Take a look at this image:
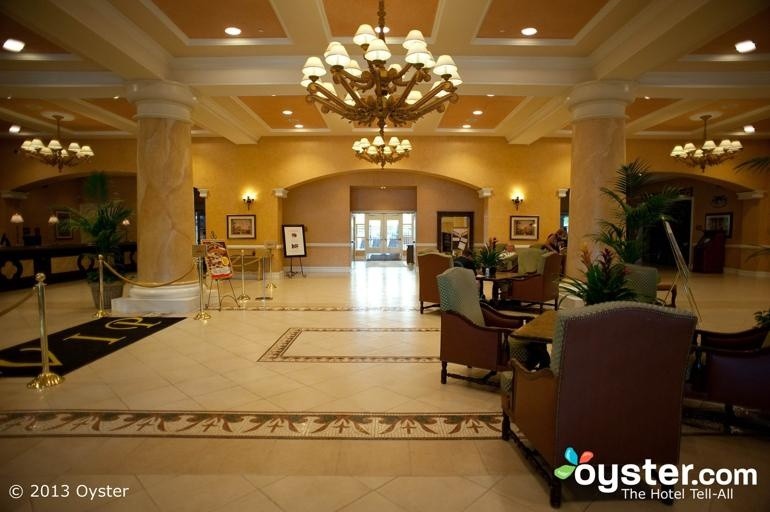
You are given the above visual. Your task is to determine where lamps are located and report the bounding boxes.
[243,192,255,211]
[122,219,130,243]
[511,192,524,211]
[298,0,462,132]
[21,112,94,173]
[670,115,743,173]
[351,120,412,169]
[47,215,59,224]
[9,213,24,247]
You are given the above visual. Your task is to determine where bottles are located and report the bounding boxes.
[486,268,489,276]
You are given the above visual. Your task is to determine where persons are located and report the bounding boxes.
[454,249,477,276]
[497,243,518,265]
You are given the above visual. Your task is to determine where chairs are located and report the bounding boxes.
[417,242,770,508]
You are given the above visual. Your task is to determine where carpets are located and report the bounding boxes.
[0,317,187,378]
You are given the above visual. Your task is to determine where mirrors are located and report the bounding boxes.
[437,211,474,253]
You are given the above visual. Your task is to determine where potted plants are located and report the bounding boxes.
[49,169,134,310]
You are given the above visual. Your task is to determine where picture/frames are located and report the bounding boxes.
[510,215,539,240]
[201,239,233,279]
[227,214,255,239]
[705,211,733,239]
[55,210,73,240]
[282,225,306,257]
